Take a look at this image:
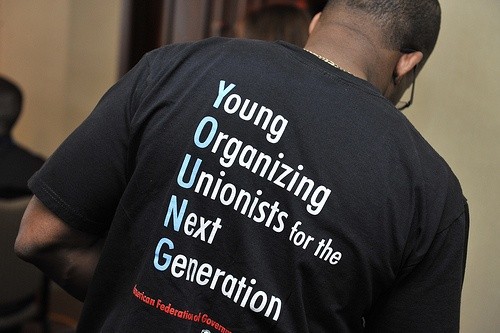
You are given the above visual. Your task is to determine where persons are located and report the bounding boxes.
[13,0,471,333]
[0,73,45,200]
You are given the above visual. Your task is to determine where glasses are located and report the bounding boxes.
[395,65,416,110]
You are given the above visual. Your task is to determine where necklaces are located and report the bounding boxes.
[301,47,355,76]
